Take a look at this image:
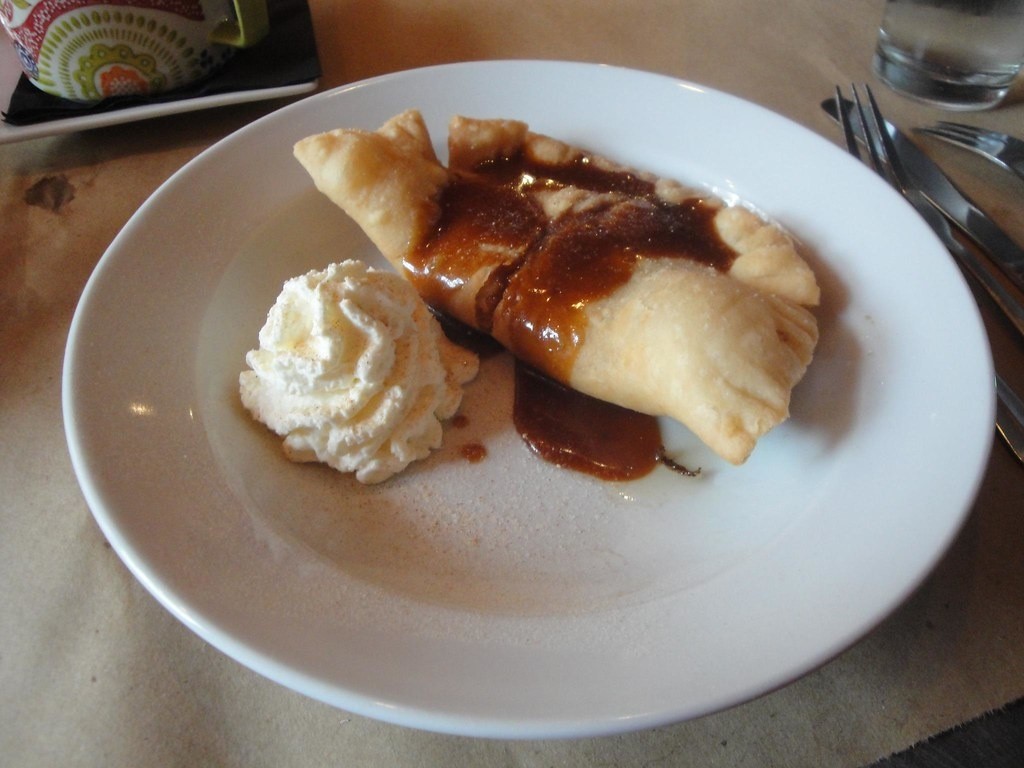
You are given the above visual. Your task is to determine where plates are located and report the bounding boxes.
[63,58,997,740]
[0,0,320,144]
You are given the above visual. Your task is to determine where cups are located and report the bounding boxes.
[871,0,1024,112]
[0,0,268,104]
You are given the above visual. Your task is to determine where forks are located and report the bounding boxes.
[909,121,1024,183]
[835,84,1024,464]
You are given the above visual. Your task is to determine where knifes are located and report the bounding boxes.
[817,99,1023,295]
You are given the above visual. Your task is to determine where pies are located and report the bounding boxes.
[293,109,822,464]
